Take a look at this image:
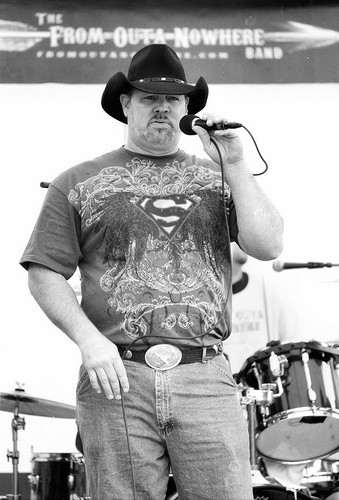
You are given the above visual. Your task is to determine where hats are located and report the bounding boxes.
[101,44,208,125]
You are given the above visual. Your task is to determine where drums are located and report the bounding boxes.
[29,450,86,500]
[238,341,339,465]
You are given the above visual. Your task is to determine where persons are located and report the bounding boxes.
[19,43,284,500]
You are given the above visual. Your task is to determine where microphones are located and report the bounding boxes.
[273,259,332,272]
[180,114,242,135]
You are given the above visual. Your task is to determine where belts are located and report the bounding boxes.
[118,345,219,371]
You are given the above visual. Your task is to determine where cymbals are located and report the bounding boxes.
[0,392,77,418]
